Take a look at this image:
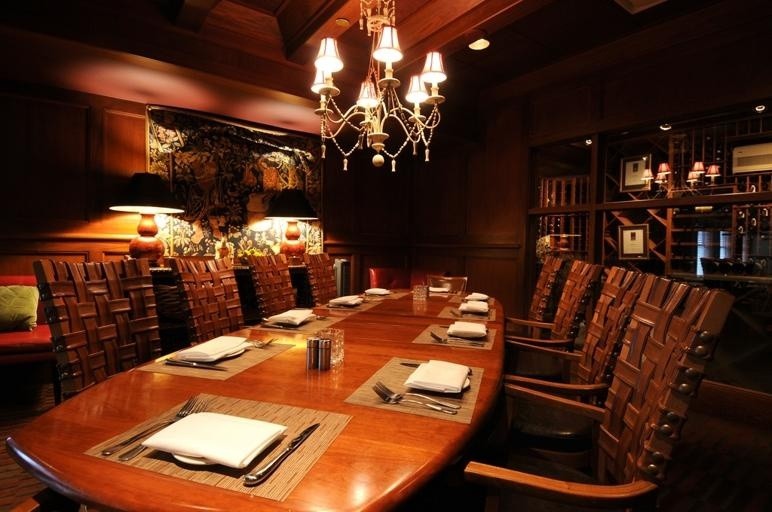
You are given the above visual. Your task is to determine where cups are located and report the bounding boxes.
[316,328,346,365]
[413,284,429,301]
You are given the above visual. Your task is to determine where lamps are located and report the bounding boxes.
[308,1,450,174]
[643,133,725,201]
[108,174,185,264]
[261,189,323,266]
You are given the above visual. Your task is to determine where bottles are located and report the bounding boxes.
[305,335,332,369]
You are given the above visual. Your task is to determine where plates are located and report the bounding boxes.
[462,376,470,388]
[170,451,215,467]
[219,347,245,359]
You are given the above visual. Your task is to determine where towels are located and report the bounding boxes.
[175,335,254,366]
[445,320,488,338]
[322,287,489,315]
[259,302,318,330]
[139,413,289,470]
[402,357,472,398]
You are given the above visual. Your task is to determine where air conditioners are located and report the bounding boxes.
[729,143,771,175]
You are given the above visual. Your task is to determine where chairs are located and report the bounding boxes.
[165,253,248,338]
[1,272,66,421]
[37,255,163,401]
[242,253,298,330]
[425,273,468,293]
[299,255,343,307]
[462,253,736,512]
[368,265,451,294]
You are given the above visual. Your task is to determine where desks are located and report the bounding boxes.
[119,258,315,356]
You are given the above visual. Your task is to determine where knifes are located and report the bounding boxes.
[260,322,305,331]
[439,325,449,328]
[165,355,230,372]
[325,304,363,310]
[400,362,472,375]
[243,422,319,486]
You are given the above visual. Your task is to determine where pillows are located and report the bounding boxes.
[2,284,41,330]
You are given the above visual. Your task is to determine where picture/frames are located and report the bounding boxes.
[618,156,651,192]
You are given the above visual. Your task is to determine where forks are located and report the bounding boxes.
[449,309,490,320]
[372,380,461,415]
[430,330,484,345]
[101,392,200,455]
[119,396,208,462]
[247,336,279,349]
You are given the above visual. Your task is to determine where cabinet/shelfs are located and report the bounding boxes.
[602,172,772,280]
